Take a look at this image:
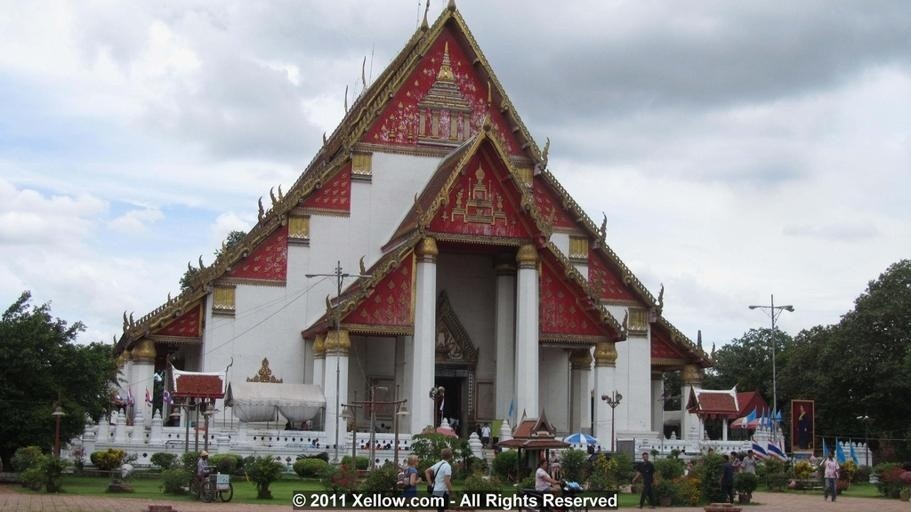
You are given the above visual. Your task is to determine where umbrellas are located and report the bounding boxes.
[563,431,598,451]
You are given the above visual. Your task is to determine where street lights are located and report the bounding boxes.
[601,390,622,452]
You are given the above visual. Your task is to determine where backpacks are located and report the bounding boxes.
[396,470,410,492]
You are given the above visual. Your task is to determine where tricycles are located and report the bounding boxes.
[188,466,233,503]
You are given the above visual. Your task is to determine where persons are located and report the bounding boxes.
[424,448,453,512]
[477,423,483,440]
[632,452,656,508]
[535,458,565,495]
[742,449,757,498]
[195,450,212,500]
[719,454,735,504]
[821,449,840,502]
[403,455,423,511]
[553,458,563,480]
[731,451,741,494]
[796,404,811,448]
[482,422,493,448]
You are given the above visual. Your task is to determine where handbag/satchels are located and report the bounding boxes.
[426,484,434,493]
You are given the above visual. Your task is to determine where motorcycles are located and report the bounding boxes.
[520,477,588,512]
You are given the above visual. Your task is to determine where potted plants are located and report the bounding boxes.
[38,454,67,493]
[656,478,673,506]
[72,446,86,470]
[732,472,757,505]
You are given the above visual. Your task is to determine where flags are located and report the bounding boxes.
[836,436,847,464]
[747,406,782,432]
[440,393,445,418]
[767,438,789,463]
[850,439,859,465]
[128,388,135,407]
[751,438,768,462]
[164,389,176,404]
[823,437,831,458]
[145,386,154,404]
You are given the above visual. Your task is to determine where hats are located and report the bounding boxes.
[200,450,208,457]
